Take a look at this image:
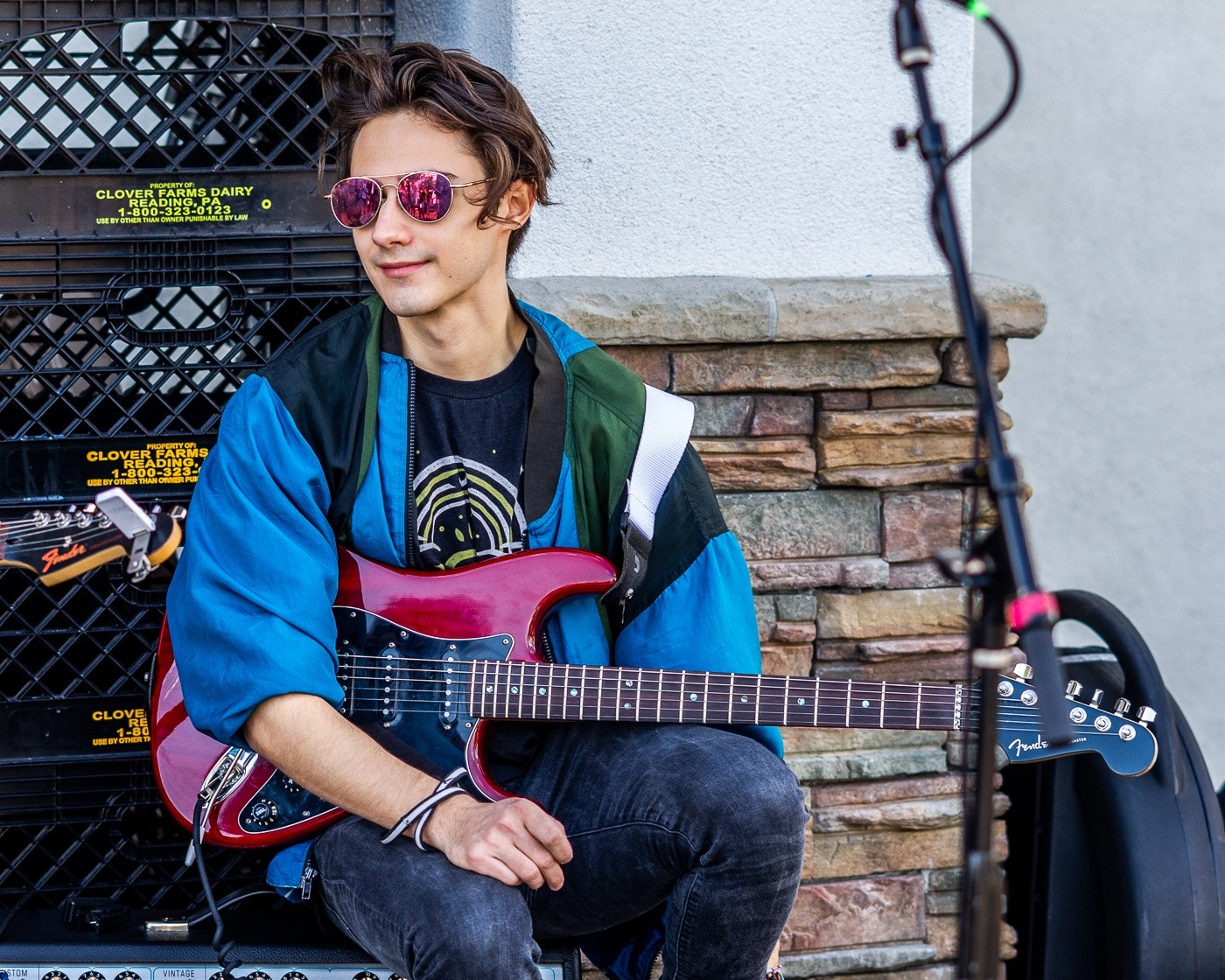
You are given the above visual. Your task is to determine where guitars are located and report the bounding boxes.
[149,544,1158,852]
[0,484,183,590]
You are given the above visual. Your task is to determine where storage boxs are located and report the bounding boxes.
[0,1,398,946]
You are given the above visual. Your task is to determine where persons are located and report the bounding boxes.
[165,41,810,980]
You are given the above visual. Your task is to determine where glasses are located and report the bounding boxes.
[324,171,498,229]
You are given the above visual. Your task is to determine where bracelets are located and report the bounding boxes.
[765,965,784,980]
[379,765,469,853]
